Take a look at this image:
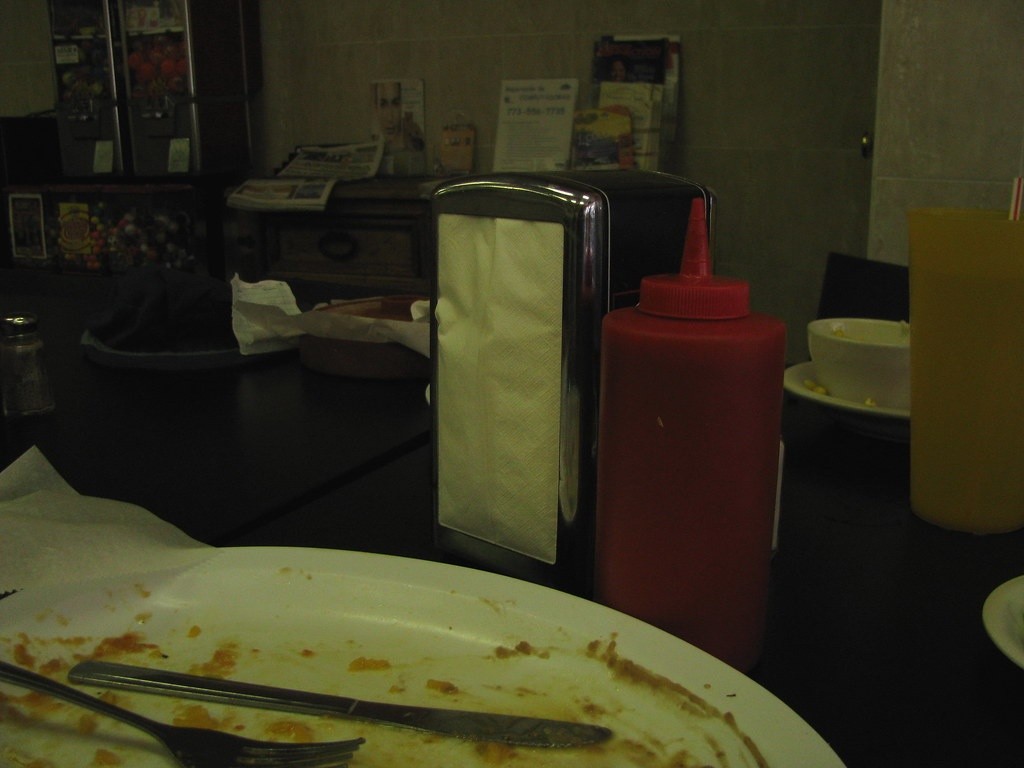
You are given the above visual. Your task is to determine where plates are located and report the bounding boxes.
[81,327,272,368]
[1,545,847,768]
[782,359,912,418]
[982,574,1024,672]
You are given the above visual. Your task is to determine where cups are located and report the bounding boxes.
[807,317,911,415]
[901,203,1024,538]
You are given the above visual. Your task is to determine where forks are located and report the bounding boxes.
[0,658,367,767]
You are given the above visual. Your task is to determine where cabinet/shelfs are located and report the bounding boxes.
[225,169,709,343]
[119,1,248,176]
[46,1,115,181]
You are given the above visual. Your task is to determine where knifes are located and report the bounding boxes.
[68,656,616,750]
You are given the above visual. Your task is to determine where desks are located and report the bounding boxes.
[1,261,1023,768]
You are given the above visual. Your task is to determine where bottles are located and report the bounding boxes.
[592,195,788,674]
[0,310,56,421]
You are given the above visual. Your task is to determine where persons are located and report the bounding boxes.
[371,82,406,155]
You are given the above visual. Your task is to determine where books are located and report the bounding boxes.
[492,35,683,177]
[437,122,476,177]
[371,77,427,177]
[227,140,383,210]
